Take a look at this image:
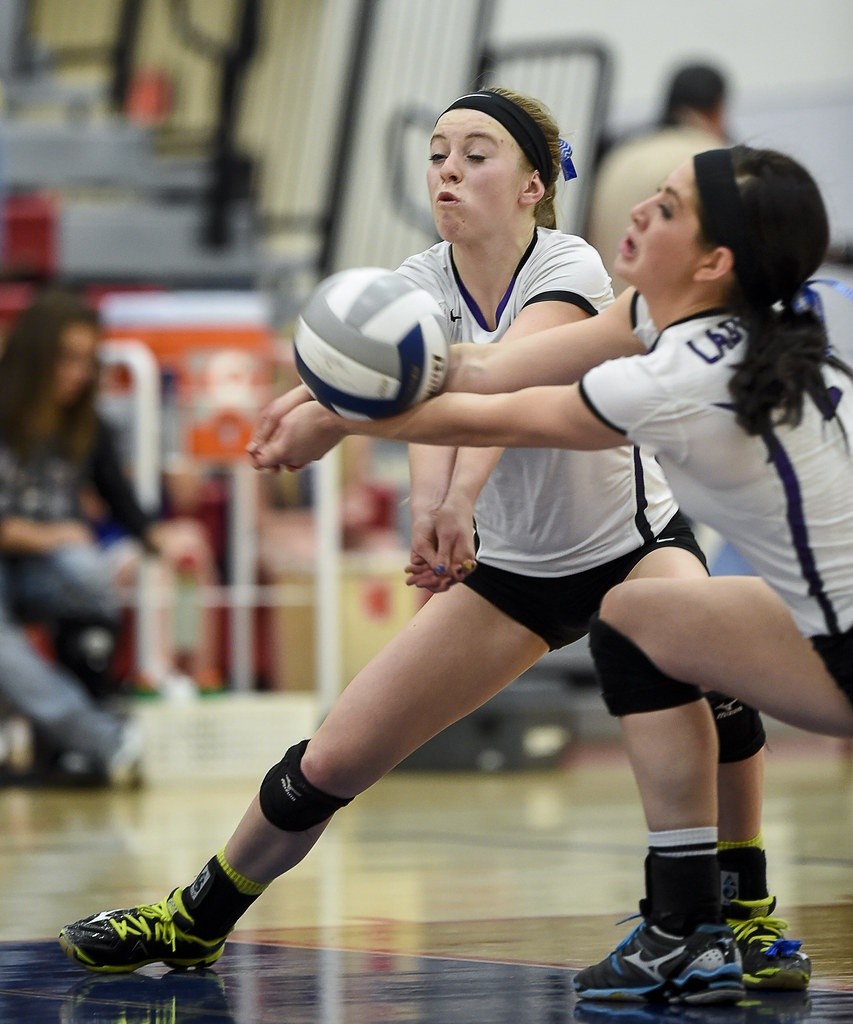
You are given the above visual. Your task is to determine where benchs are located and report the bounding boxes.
[0,80,410,559]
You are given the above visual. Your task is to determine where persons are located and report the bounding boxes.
[1,293,196,790]
[591,63,745,305]
[54,85,814,998]
[244,145,853,1012]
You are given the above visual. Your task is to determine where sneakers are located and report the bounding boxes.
[570,898,746,1006]
[720,896,812,994]
[60,970,227,1024]
[56,886,238,972]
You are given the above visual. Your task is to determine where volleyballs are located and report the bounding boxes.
[290,265,451,424]
